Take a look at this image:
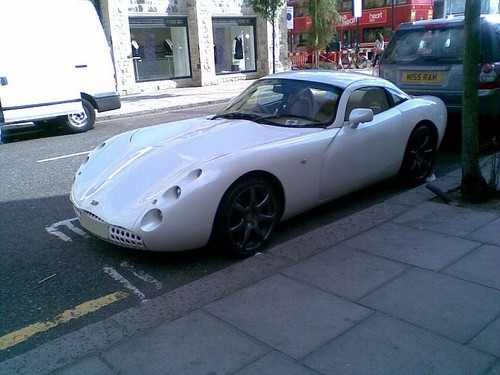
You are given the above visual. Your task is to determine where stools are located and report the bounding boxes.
[164,54,173,75]
[133,56,142,81]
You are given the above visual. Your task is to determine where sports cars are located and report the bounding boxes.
[67,67,448,260]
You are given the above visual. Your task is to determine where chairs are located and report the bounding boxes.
[315,100,336,123]
[362,89,389,110]
[288,88,315,119]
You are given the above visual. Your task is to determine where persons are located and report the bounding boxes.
[373,32,384,66]
[234,36,243,59]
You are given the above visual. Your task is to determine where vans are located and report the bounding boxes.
[0,0,121,137]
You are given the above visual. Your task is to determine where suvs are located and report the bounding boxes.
[368,13,500,156]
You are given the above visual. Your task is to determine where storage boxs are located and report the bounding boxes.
[231,65,239,71]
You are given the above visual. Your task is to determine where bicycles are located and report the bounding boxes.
[341,40,368,70]
